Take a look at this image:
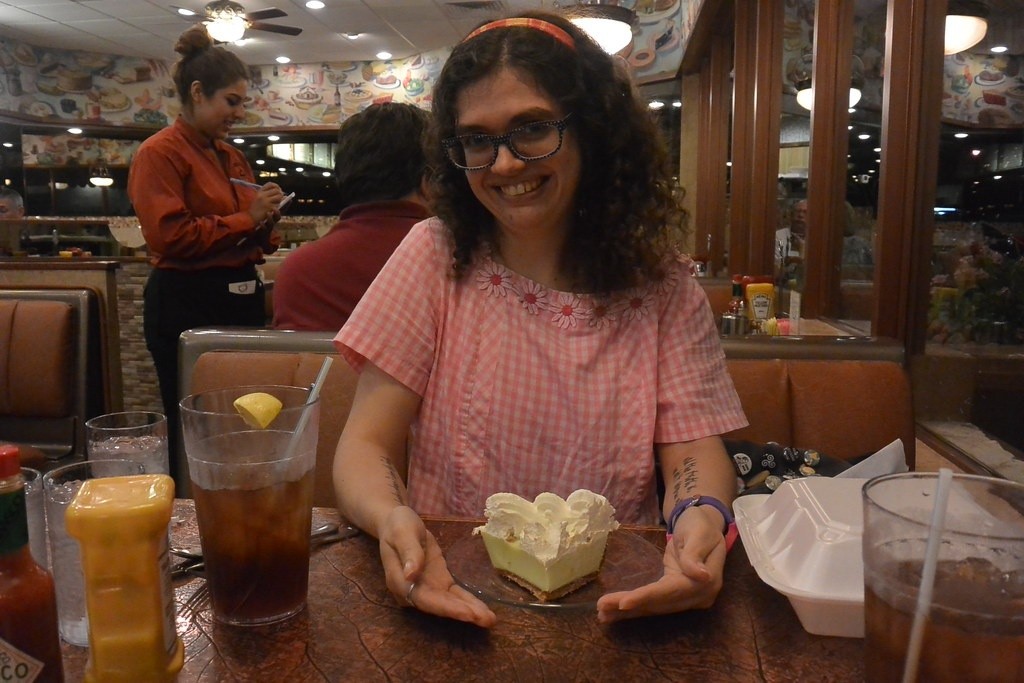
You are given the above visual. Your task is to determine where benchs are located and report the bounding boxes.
[178,327,916,509]
[274,248,292,258]
[0,285,107,476]
[261,256,286,313]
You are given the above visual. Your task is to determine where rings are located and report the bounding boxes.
[406,580,417,609]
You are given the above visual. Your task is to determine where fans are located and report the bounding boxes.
[168,0,303,37]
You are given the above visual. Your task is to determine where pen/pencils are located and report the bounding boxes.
[230,177,287,195]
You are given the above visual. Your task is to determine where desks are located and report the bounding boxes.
[60,497,1024,683]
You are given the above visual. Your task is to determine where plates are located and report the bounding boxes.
[446,526,665,609]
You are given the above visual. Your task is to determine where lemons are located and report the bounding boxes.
[234,393,283,429]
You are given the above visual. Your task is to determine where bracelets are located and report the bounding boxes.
[665,493,739,556]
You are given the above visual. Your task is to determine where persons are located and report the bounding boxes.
[273,101,437,336]
[331,14,749,627]
[774,198,872,285]
[0,186,37,255]
[129,28,285,500]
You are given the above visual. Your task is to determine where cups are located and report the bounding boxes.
[180,385,321,628]
[21,458,145,683]
[86,411,169,478]
[862,470,1024,683]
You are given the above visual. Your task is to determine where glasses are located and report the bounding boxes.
[442,111,574,170]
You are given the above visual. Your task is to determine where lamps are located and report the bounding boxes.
[794,78,864,112]
[88,156,114,187]
[47,168,70,191]
[559,3,635,55]
[200,17,247,43]
[943,0,990,58]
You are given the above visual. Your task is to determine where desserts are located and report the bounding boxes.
[472,489,618,602]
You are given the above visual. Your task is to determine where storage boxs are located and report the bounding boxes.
[727,471,1016,642]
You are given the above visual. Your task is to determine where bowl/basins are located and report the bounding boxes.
[731,476,864,638]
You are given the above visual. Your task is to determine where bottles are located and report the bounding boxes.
[720,273,800,340]
[0,444,62,682]
[64,475,184,682]
